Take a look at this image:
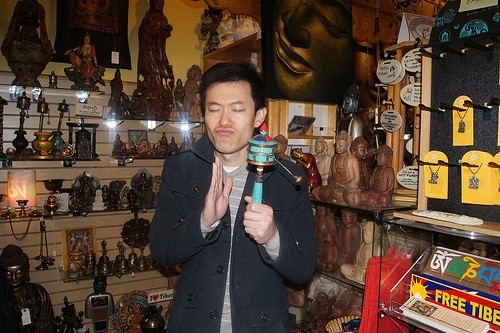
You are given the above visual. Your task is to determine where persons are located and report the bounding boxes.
[289,148,323,195]
[70,171,151,211]
[313,137,332,185]
[64,31,104,80]
[137,0,174,90]
[183,64,202,113]
[306,276,364,333]
[127,305,143,333]
[338,82,364,141]
[272,134,291,162]
[148,60,318,333]
[271,0,353,102]
[312,130,361,207]
[85,273,114,318]
[312,206,383,286]
[109,68,128,105]
[361,143,395,208]
[173,78,185,112]
[0,244,56,333]
[350,136,372,194]
[113,132,180,157]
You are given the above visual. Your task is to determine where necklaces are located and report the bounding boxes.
[468,163,483,189]
[428,165,442,185]
[458,107,469,133]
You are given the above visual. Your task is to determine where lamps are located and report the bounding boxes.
[7,168,37,218]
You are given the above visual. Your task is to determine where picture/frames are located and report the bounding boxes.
[288,114,317,137]
[61,226,97,272]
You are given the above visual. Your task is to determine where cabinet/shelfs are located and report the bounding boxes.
[267,100,337,187]
[286,193,417,333]
[373,206,500,333]
[0,81,207,285]
[201,32,269,137]
[381,40,430,204]
